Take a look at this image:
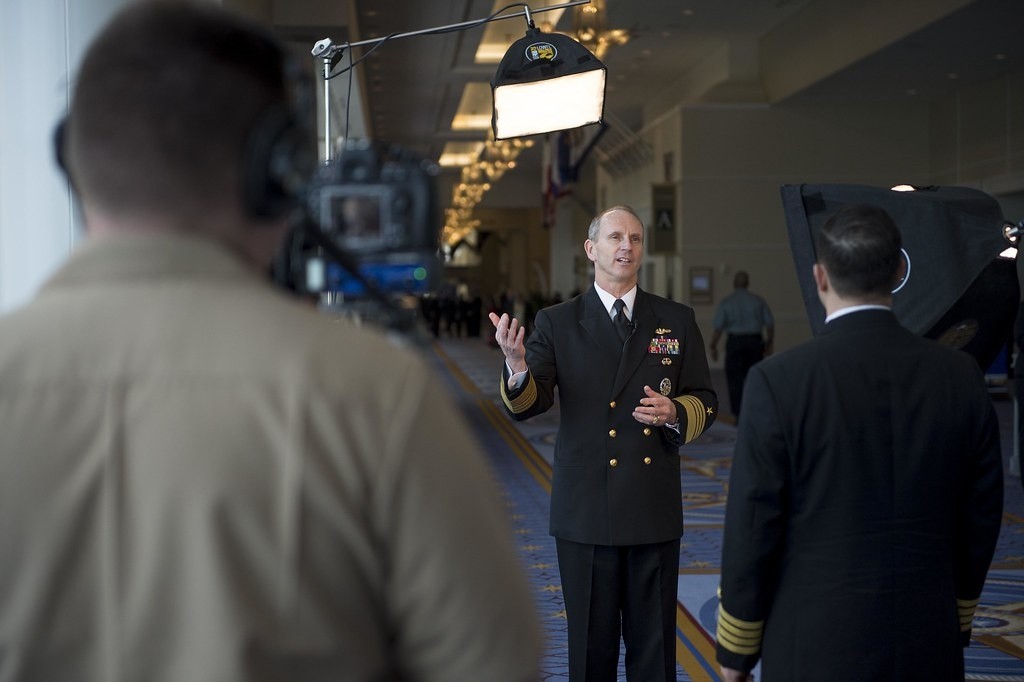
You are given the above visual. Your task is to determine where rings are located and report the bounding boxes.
[654,416,659,424]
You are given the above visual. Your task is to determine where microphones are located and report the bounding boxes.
[625,320,638,335]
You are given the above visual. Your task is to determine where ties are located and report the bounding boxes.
[613,298,633,343]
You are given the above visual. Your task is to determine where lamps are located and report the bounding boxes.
[313,0,609,304]
[777,175,1023,347]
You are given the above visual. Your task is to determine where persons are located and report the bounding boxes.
[0,0,548,682]
[710,271,775,425]
[420,287,581,347]
[717,204,1005,682]
[488,205,722,682]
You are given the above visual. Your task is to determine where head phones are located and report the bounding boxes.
[53,23,320,223]
